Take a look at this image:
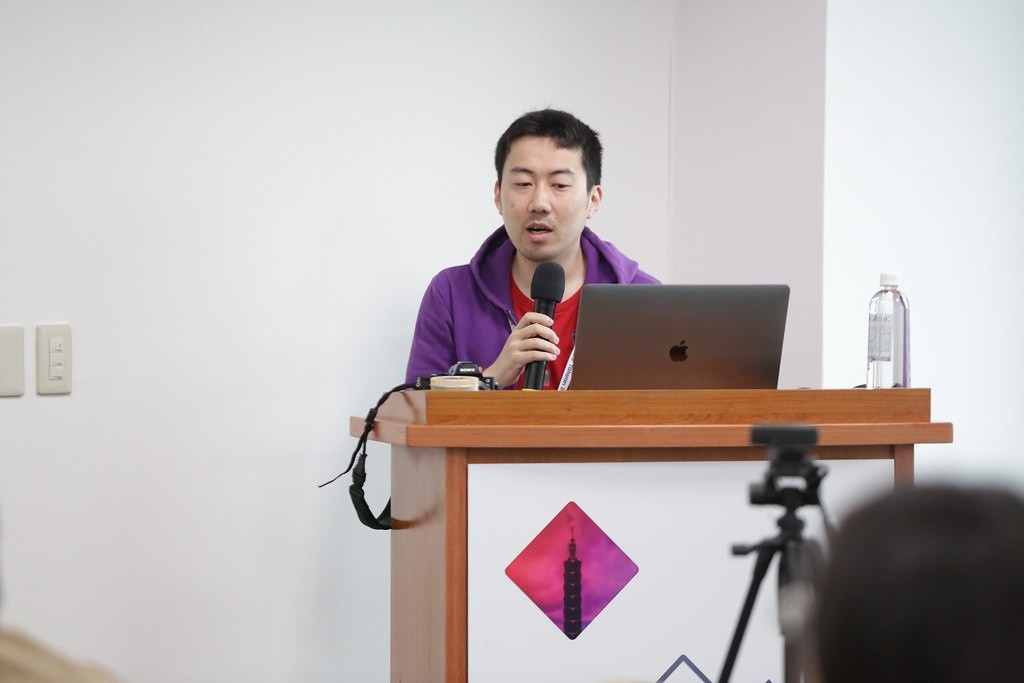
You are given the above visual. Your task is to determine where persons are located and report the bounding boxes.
[799,484,1023,683]
[405,110,662,392]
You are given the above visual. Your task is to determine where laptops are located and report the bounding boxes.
[569,284,790,391]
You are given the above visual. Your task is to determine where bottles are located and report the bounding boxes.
[866,272,910,389]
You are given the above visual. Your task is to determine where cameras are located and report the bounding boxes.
[416,361,499,390]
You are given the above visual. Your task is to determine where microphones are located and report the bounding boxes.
[522,262,565,390]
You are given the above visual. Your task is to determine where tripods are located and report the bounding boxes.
[717,424,829,683]
